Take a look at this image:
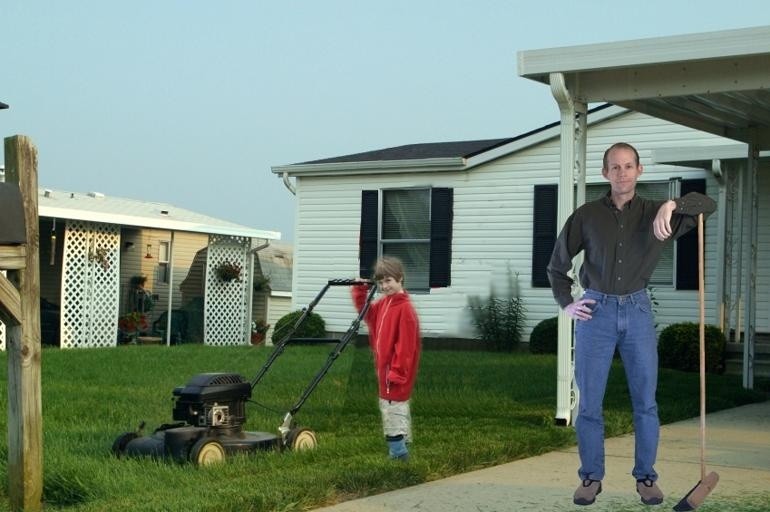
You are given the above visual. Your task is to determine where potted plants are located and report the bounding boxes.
[251,319,270,343]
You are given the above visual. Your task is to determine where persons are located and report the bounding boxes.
[350,258,422,462]
[545,141,716,504]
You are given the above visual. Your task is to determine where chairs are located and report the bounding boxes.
[152,309,187,344]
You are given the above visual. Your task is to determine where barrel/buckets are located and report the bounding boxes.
[162,310,187,345]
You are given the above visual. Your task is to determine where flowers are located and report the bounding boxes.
[118,312,149,334]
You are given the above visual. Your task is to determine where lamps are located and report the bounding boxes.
[144,244,152,258]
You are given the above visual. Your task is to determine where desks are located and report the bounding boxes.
[136,337,162,344]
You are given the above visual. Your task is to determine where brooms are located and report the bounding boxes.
[672,211,720,512]
